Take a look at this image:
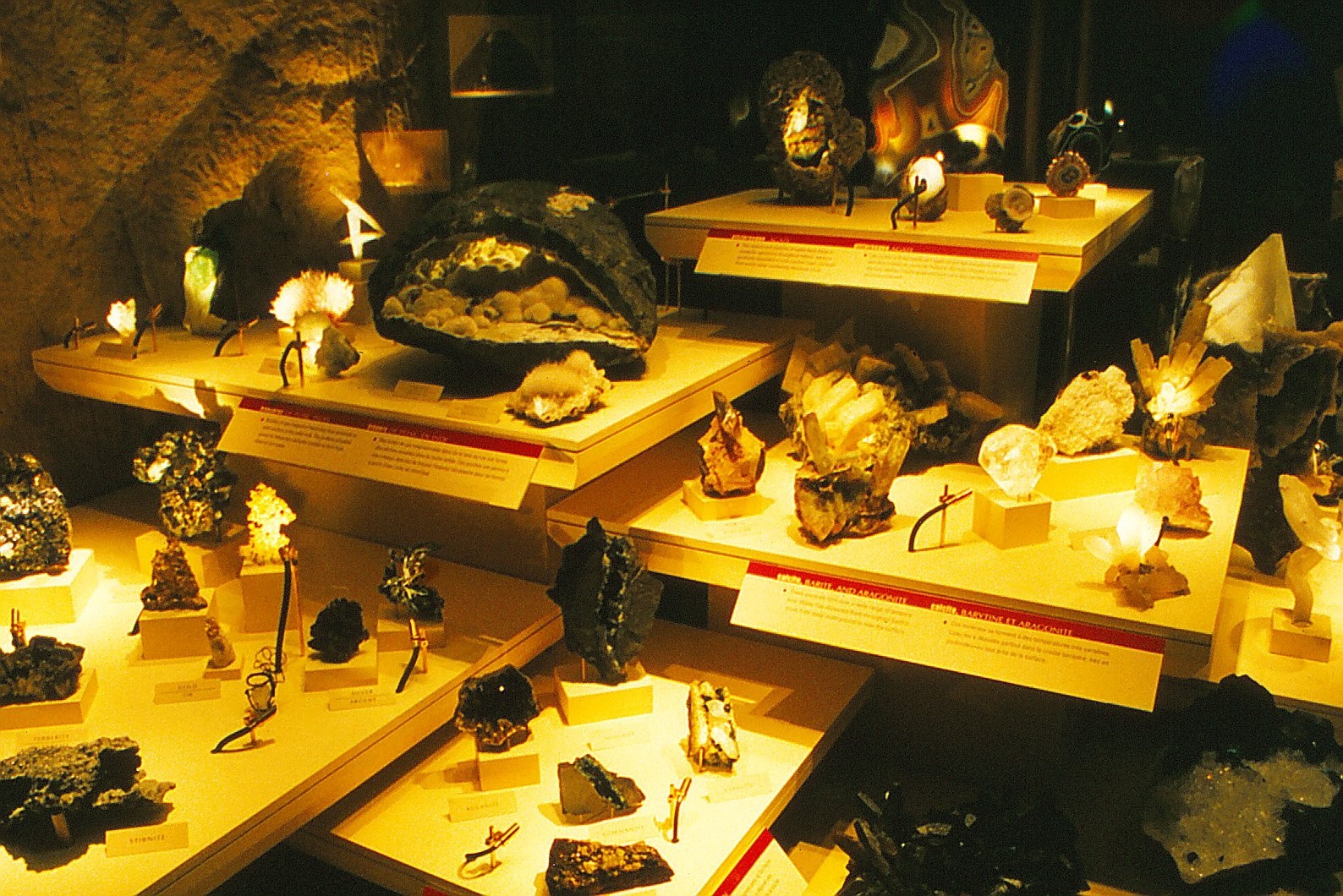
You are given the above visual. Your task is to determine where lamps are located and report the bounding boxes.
[450,27,556,183]
